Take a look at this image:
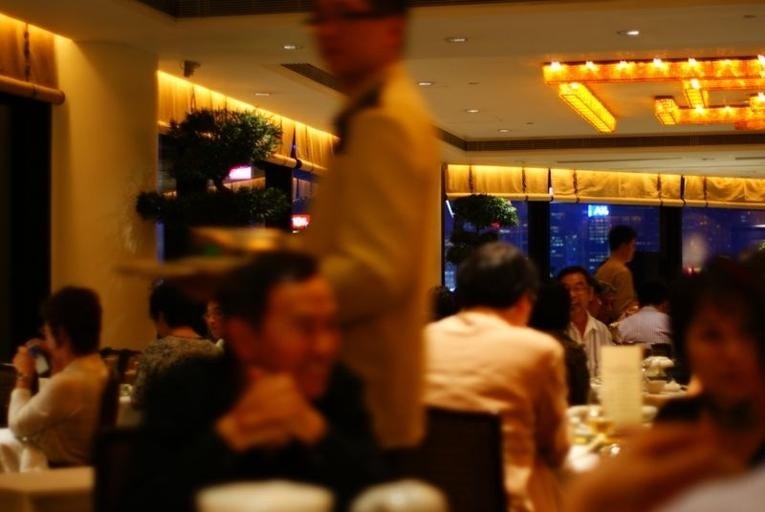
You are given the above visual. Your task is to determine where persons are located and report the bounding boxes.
[262,4,441,460]
